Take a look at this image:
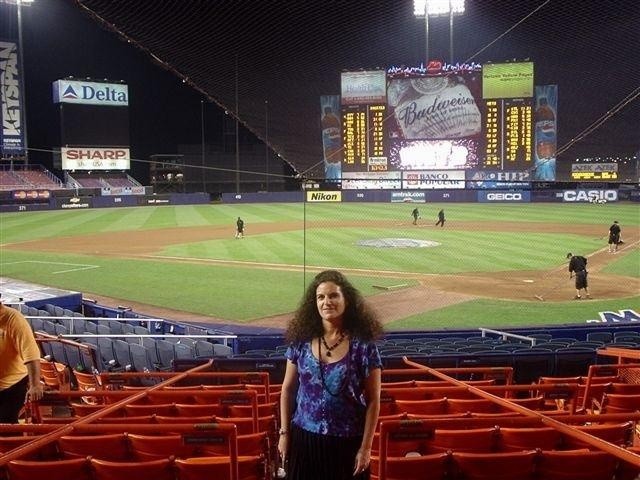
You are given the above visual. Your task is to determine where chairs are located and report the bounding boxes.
[2,301,638,479]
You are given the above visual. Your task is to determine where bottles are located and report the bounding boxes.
[321,105,342,164]
[76,363,83,374]
[536,98,557,159]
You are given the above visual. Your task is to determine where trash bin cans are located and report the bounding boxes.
[123,187,131,195]
[236,195,241,199]
[101,188,111,195]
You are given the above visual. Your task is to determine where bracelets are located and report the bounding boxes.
[279,426,290,435]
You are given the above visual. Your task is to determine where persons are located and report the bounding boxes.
[410,206,422,225]
[435,208,446,227]
[607,220,622,253]
[278,269,384,480]
[566,251,592,301]
[234,216,245,239]
[0,300,44,424]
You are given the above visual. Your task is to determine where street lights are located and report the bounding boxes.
[413,0,465,67]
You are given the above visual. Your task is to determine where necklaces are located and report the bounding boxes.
[319,333,354,398]
